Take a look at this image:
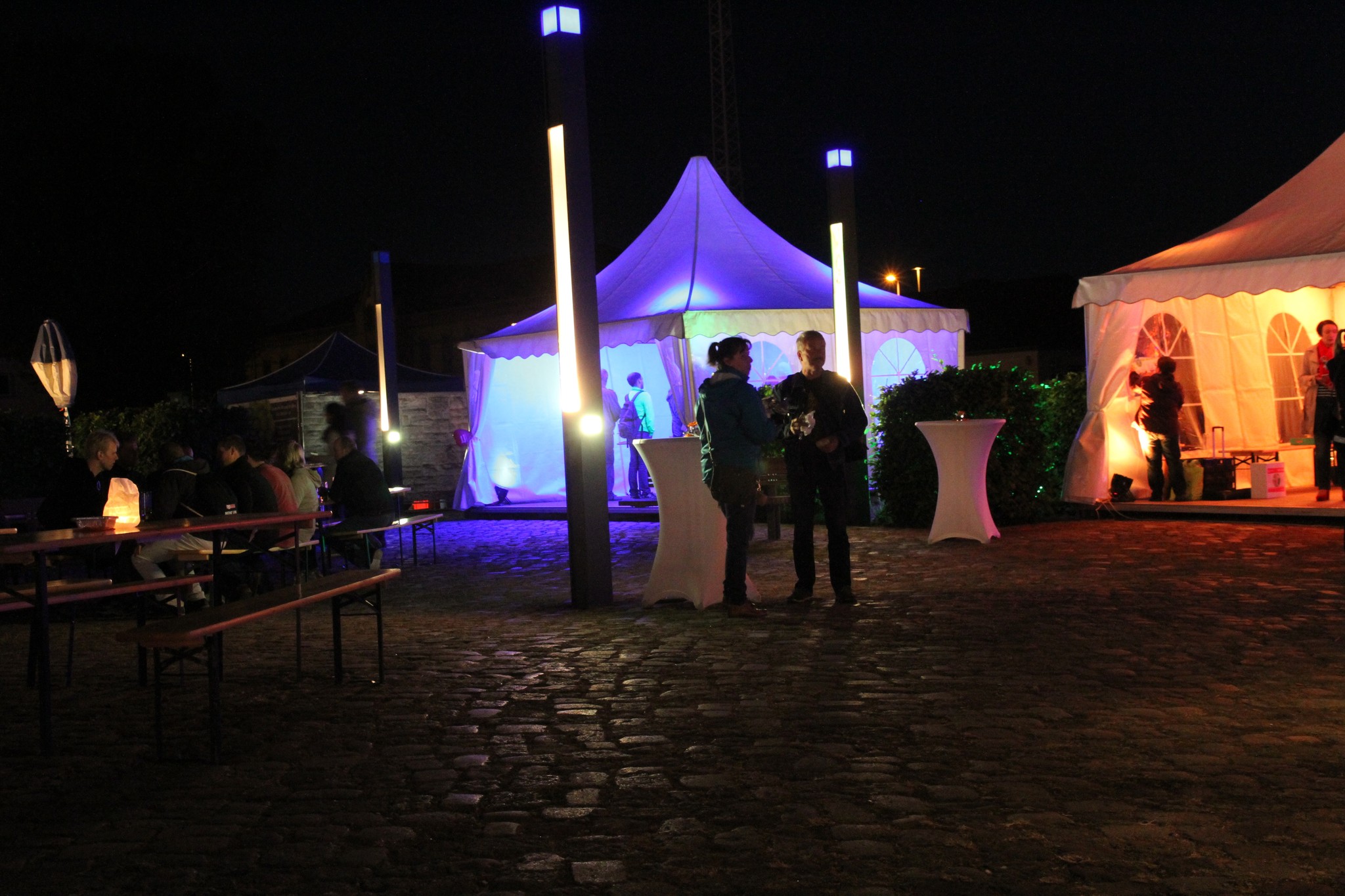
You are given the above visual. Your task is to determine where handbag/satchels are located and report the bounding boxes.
[1183,460,1204,500]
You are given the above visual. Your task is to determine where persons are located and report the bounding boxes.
[1299,321,1345,501]
[246,446,301,549]
[601,369,621,501]
[328,436,393,570]
[132,435,277,619]
[667,390,685,437]
[321,380,378,476]
[40,430,144,584]
[772,331,868,603]
[1129,357,1195,502]
[275,441,321,543]
[626,372,656,498]
[695,337,782,618]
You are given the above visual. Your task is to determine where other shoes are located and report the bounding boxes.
[1174,497,1185,501]
[1149,492,1162,501]
[835,596,857,604]
[608,495,622,501]
[722,598,769,618]
[788,592,813,601]
[1316,489,1329,502]
[642,492,656,498]
[159,605,184,619]
[1342,490,1345,500]
[631,493,640,499]
[184,598,209,611]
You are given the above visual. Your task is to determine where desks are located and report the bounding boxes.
[0,509,333,688]
[914,415,1008,547]
[319,486,412,565]
[1218,441,1337,488]
[631,432,765,610]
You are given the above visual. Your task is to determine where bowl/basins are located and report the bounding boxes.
[71,516,117,530]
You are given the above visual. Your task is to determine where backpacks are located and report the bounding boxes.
[618,391,646,441]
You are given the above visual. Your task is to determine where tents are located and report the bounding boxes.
[451,157,969,513]
[1062,130,1345,516]
[220,333,466,493]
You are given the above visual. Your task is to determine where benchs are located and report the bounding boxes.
[331,513,444,568]
[113,568,401,765]
[0,572,213,652]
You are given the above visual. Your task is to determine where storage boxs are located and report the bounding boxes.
[1250,462,1288,499]
